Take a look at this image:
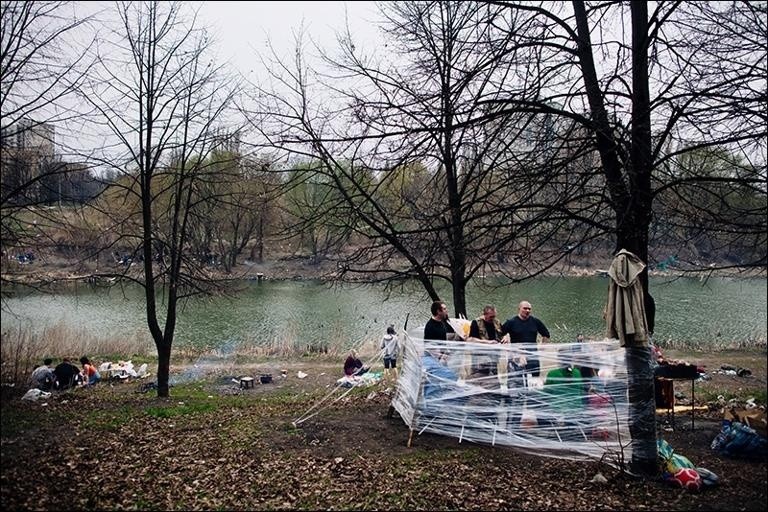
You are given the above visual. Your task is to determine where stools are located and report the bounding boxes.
[241,377,255,389]
[546,416,589,442]
[458,413,498,447]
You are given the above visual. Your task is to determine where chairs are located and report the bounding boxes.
[416,371,445,437]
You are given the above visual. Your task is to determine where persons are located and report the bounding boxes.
[28,356,101,391]
[504,353,528,433]
[465,356,499,426]
[536,345,603,440]
[380,327,401,367]
[654,342,664,364]
[495,300,551,426]
[344,349,370,375]
[465,305,511,404]
[424,301,468,416]
